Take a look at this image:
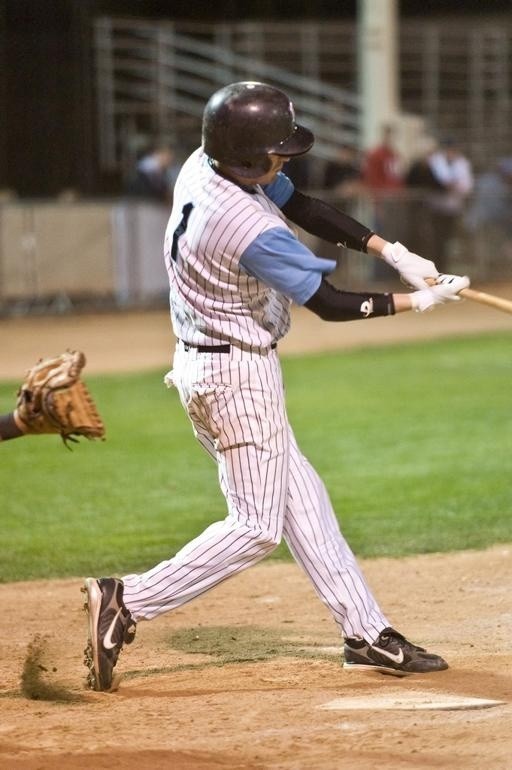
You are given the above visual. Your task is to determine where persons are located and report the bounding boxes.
[0,349,106,452]
[80,80,471,692]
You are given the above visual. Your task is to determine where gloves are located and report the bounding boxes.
[381,240,440,293]
[409,272,472,313]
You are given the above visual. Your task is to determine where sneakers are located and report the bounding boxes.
[342,626,450,677]
[80,576,137,693]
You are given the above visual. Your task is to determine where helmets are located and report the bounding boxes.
[199,79,316,179]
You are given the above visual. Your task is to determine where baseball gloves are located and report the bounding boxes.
[15,349,105,453]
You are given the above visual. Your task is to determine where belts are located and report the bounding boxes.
[175,339,280,355]
